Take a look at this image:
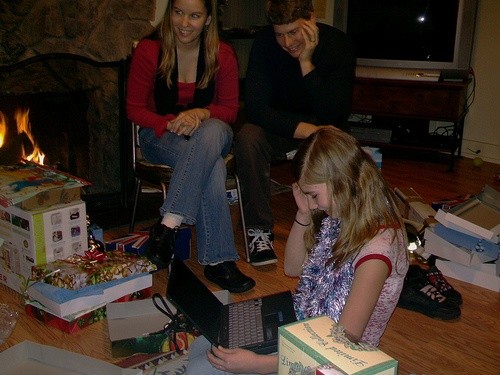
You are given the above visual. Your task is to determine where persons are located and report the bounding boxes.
[187,128,408,375]
[235,0,357,267]
[126,0,256,294]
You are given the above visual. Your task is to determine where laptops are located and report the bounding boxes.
[165,253,297,355]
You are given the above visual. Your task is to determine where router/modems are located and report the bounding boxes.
[440,71,464,82]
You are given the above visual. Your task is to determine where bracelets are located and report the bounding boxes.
[294,218,311,226]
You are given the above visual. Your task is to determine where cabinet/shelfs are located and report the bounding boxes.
[349,77,472,168]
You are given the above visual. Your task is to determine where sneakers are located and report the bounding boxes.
[243,228,278,266]
[397,261,464,320]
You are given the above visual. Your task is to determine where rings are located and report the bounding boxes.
[311,39,316,42]
[218,365,223,370]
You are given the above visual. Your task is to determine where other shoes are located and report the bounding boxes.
[138,218,180,268]
[204,260,256,293]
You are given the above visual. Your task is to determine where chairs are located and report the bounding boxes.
[127,122,251,263]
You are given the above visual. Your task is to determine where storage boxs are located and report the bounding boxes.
[106,290,233,359]
[104,226,192,274]
[277,315,398,375]
[0,201,87,294]
[424,184,500,292]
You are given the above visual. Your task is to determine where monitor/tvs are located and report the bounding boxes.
[333,0,479,71]
